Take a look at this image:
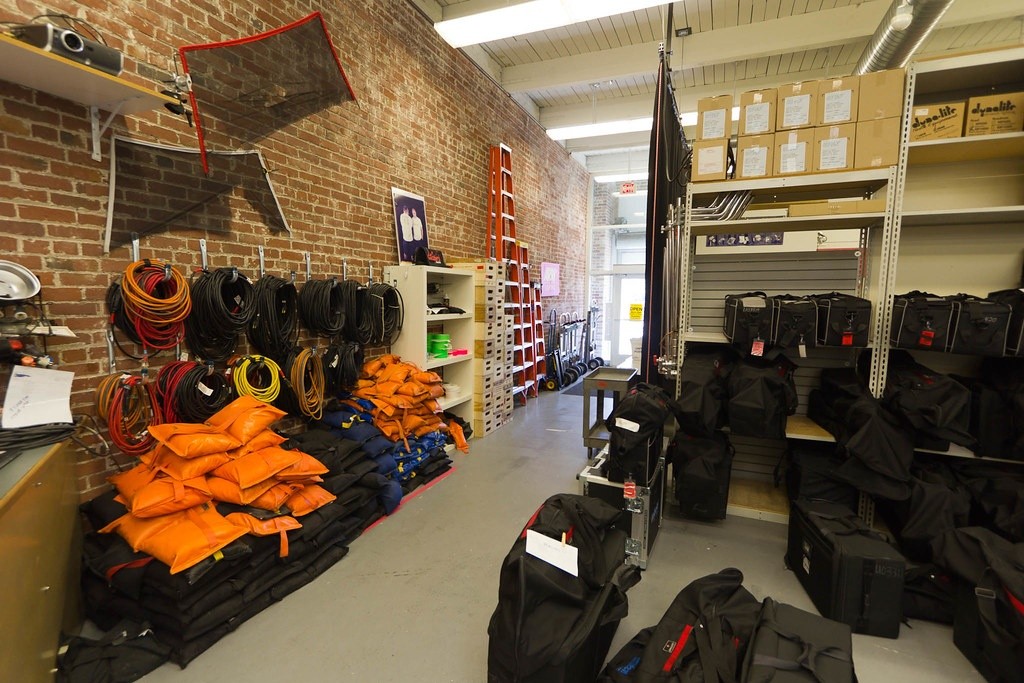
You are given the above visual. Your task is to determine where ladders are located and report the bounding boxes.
[516,238,549,398]
[485,142,527,407]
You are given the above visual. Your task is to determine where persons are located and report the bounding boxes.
[401,206,423,261]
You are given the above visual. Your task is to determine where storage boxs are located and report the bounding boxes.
[787,198,887,217]
[910,99,965,142]
[689,68,903,182]
[965,91,1024,137]
[444,251,515,439]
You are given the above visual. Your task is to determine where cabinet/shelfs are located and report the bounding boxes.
[582,365,639,450]
[855,47,1023,548]
[381,264,476,454]
[661,163,899,536]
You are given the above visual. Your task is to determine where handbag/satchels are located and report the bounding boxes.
[785,494,906,639]
[486,493,761,683]
[740,603,859,683]
[873,460,1024,683]
[600,288,1024,499]
